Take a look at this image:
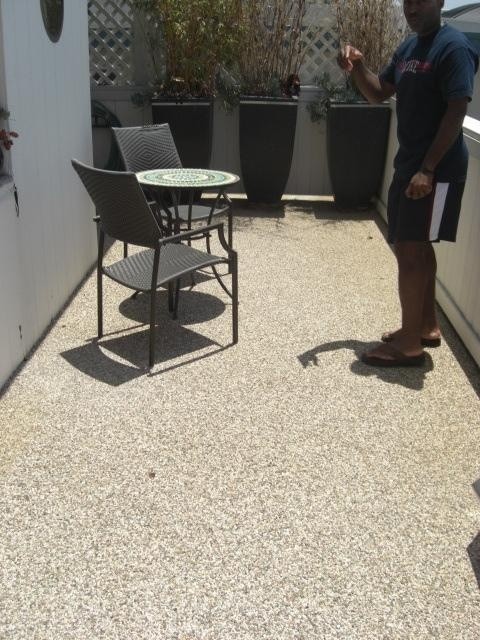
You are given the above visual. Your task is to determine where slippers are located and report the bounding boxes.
[382,329,442,347]
[361,342,427,369]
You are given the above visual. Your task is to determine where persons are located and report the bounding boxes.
[337,0,478,369]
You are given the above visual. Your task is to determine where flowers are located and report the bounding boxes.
[0,102,20,152]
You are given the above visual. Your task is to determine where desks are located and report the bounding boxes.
[133,165,241,322]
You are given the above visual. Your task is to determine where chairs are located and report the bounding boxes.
[106,122,233,276]
[70,154,242,368]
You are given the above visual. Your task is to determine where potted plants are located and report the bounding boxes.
[239,1,321,205]
[180,192,201,204]
[132,1,255,167]
[307,0,409,212]
